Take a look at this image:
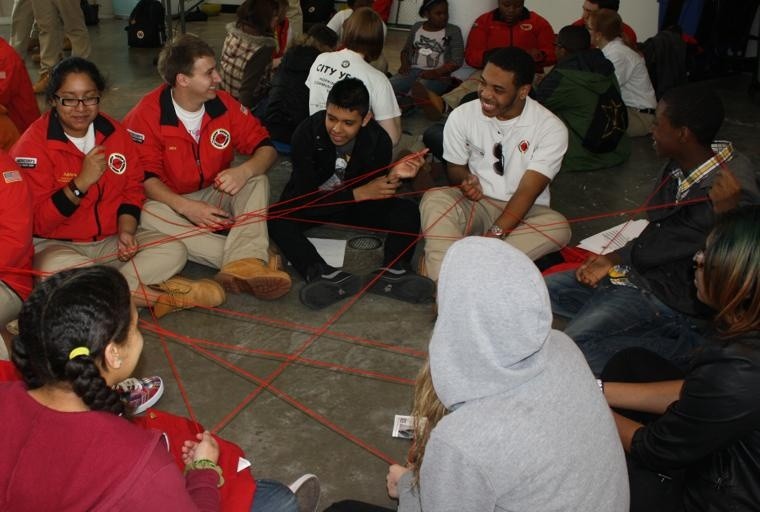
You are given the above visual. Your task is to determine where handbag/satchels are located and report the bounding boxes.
[125,0,166,48]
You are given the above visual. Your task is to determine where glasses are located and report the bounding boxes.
[493,142,504,176]
[55,94,99,105]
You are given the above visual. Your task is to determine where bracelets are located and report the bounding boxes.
[595,378,604,395]
[184,459,224,491]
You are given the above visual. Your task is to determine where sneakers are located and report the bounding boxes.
[411,81,444,121]
[365,262,435,304]
[111,376,164,416]
[32,73,52,94]
[215,241,292,300]
[148,275,227,319]
[300,266,358,311]
[288,473,320,512]
[27,38,72,62]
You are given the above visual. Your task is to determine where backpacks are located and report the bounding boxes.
[561,79,628,155]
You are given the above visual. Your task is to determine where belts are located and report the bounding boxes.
[639,108,655,115]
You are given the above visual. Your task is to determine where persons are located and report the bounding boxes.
[594,204,760,512]
[319,236,630,512]
[0,265,321,511]
[0,1,759,383]
